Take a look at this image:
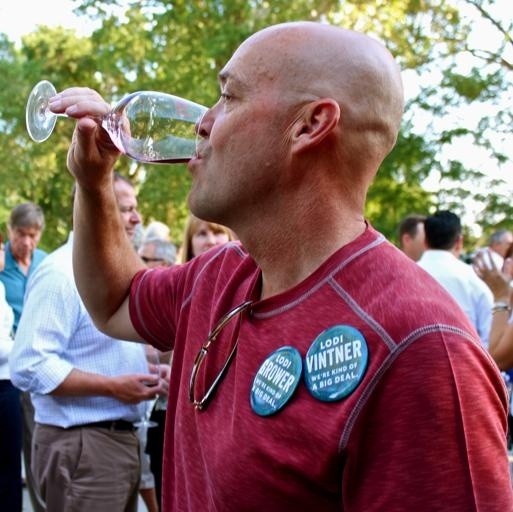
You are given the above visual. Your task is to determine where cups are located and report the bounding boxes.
[188,299,258,412]
[141,256,165,262]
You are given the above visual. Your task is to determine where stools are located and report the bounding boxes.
[88,419,136,433]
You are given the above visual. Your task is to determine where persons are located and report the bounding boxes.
[0,204,48,512]
[398,212,513,456]
[47,21,513,512]
[10,180,171,511]
[132,227,178,512]
[184,218,232,261]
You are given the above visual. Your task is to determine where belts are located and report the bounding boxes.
[118,344,161,428]
[25,79,210,166]
